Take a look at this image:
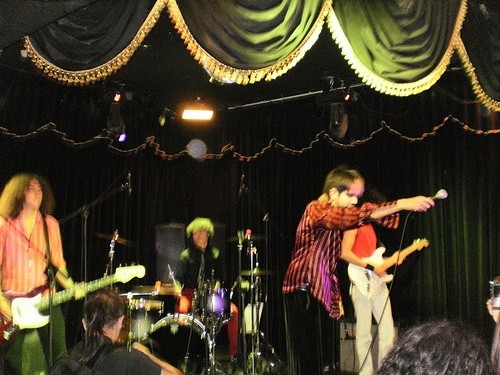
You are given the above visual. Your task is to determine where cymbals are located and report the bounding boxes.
[240,266,270,277]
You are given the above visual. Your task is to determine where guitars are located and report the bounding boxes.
[0,263,146,341]
[348,237,429,297]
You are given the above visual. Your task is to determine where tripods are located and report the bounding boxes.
[233,229,288,375]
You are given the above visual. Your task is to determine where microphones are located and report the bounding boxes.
[431,188,448,202]
[127,170,132,193]
[240,173,245,193]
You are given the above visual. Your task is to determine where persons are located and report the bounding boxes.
[373,319,496,375]
[47,290,182,375]
[340,185,403,374]
[173,216,242,375]
[2,173,86,374]
[281,167,436,375]
[486,277,500,374]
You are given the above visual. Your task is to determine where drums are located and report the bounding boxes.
[118,291,164,342]
[150,313,211,360]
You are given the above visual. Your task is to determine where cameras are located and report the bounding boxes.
[489,280,500,308]
